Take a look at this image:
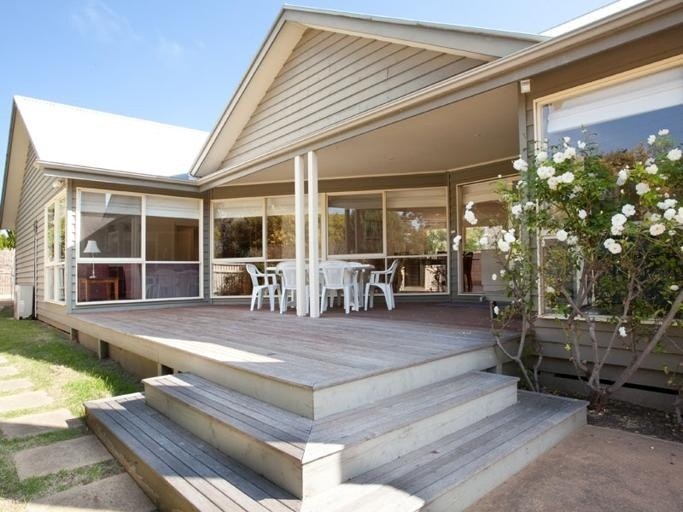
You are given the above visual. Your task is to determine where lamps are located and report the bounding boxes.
[51,180,64,190]
[83,240,101,279]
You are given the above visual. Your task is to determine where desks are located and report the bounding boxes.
[78,277,120,302]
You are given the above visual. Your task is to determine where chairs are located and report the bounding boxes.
[145,268,197,299]
[244,258,400,315]
[462,251,473,292]
